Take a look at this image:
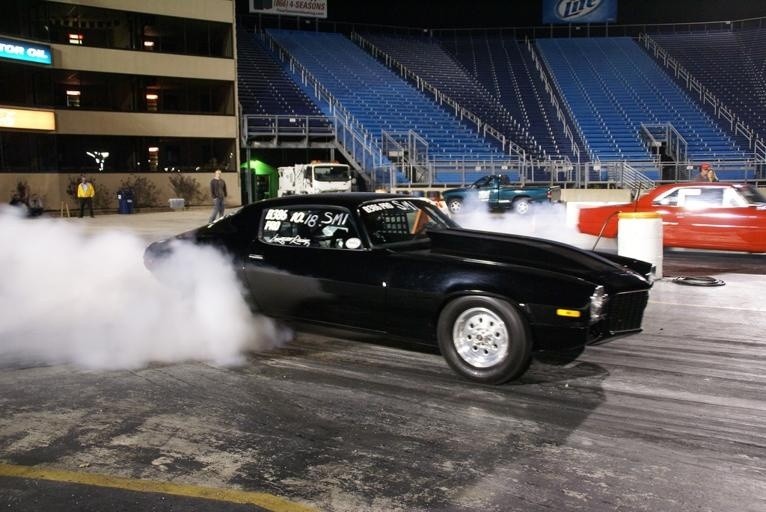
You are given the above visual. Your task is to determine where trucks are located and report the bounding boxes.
[277,162,355,196]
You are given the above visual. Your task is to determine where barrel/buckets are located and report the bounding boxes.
[617,212,663,280]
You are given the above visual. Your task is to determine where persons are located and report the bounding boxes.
[8,180,43,217]
[695,163,719,182]
[208,170,228,222]
[76,176,96,218]
[629,189,637,202]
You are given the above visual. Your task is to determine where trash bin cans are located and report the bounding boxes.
[117,190,135,214]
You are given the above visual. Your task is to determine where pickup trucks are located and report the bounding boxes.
[442,173,562,215]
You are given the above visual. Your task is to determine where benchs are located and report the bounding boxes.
[236,25,765,184]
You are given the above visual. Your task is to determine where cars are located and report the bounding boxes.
[578,182,766,253]
[143,192,655,385]
